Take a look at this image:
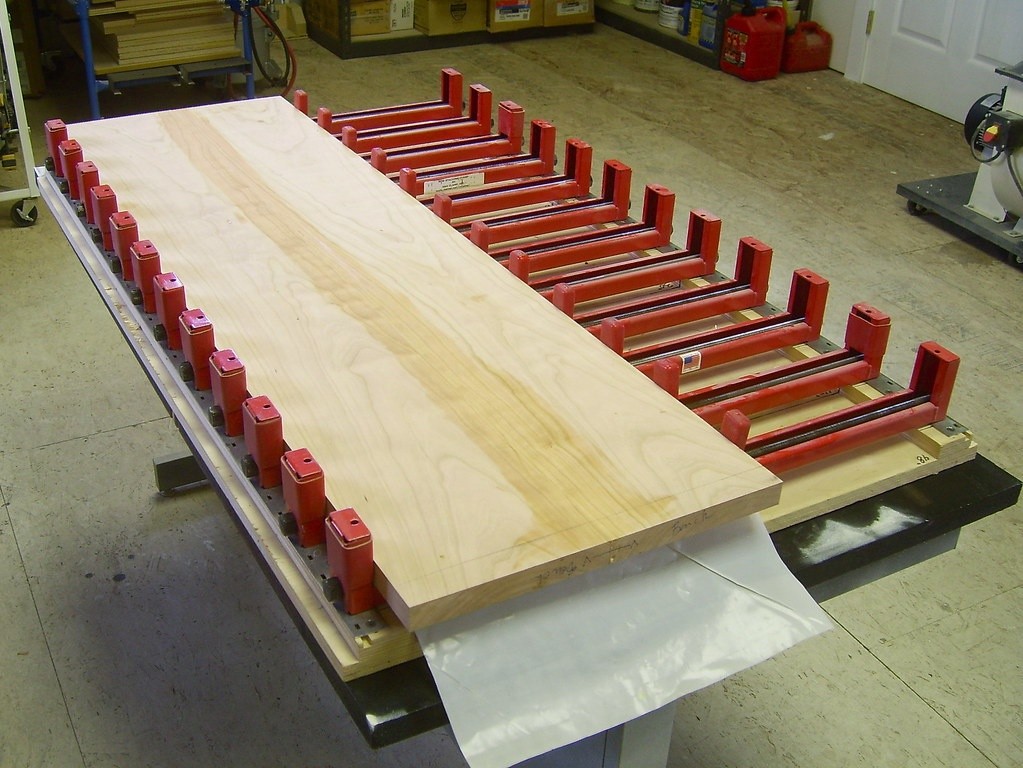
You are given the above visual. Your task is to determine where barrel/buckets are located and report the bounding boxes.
[780,21,833,73]
[720,4,787,81]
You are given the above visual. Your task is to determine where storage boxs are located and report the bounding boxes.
[306,0,594,36]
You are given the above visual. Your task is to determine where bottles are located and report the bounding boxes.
[677,3,691,36]
[698,3,718,48]
